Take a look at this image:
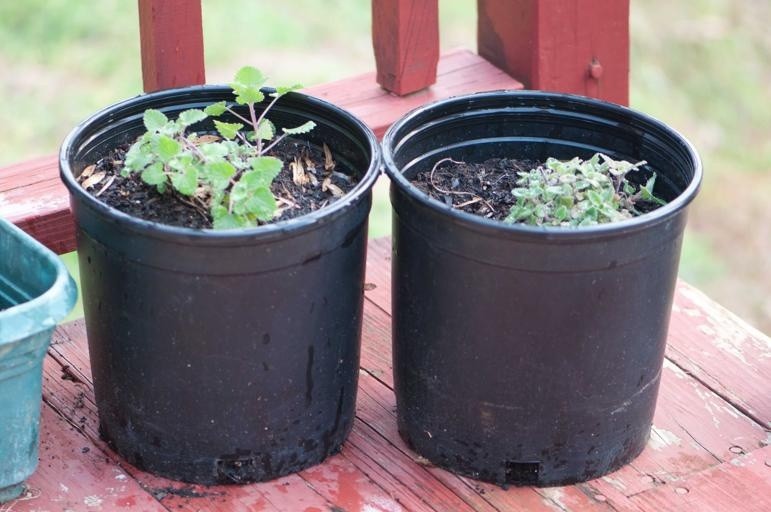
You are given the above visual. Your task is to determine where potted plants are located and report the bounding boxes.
[381,90,703,488]
[60,65,382,483]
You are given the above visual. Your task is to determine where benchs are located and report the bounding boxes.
[0,1,770,512]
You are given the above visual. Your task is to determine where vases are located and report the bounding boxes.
[0,219,78,507]
